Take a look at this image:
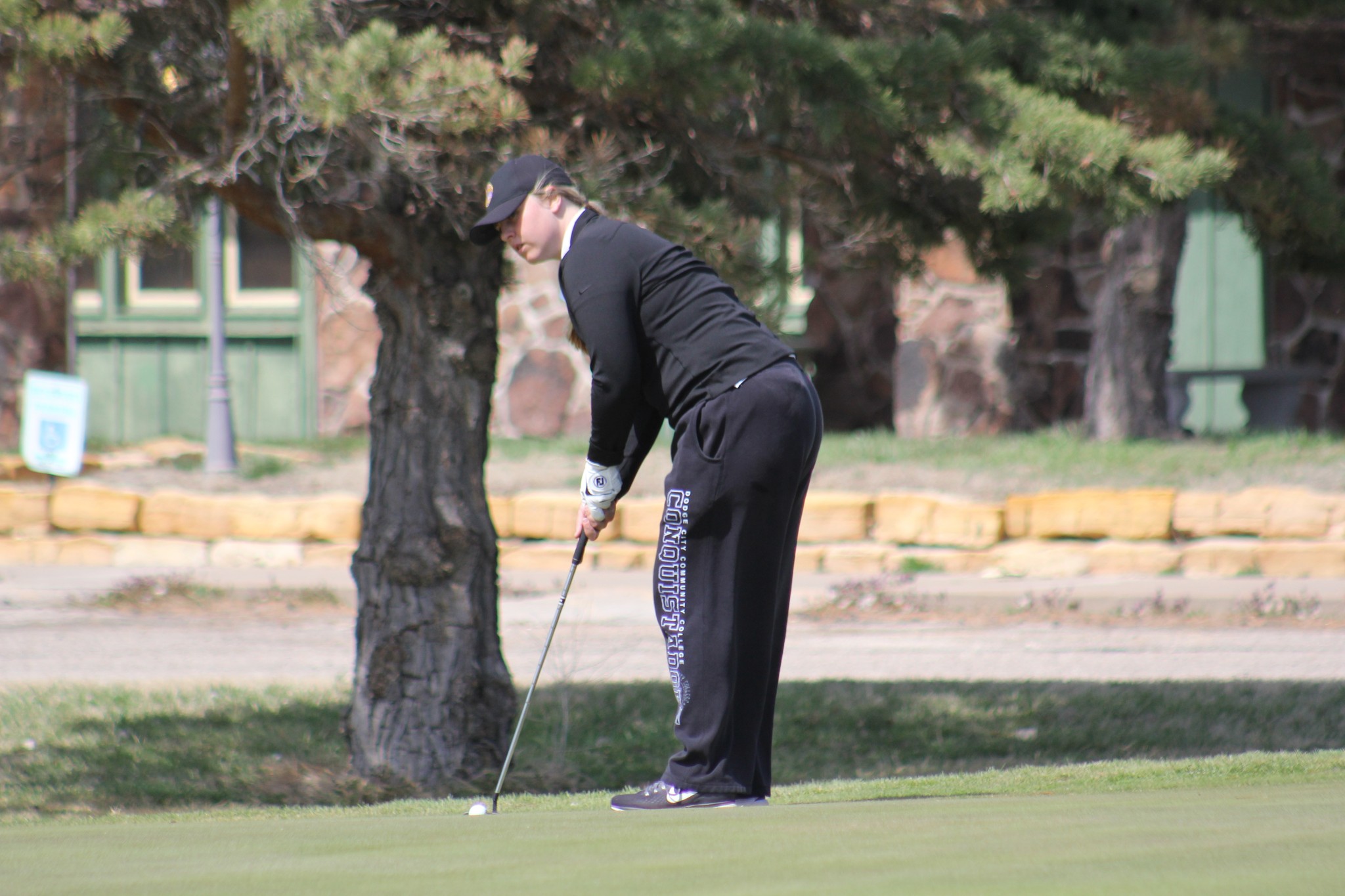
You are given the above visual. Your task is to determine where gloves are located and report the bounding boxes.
[579,458,622,522]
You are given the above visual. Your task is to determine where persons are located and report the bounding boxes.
[471,156,824,813]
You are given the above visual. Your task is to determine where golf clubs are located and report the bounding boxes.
[458,525,589,816]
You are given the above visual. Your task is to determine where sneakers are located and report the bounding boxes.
[733,793,769,806]
[610,780,737,811]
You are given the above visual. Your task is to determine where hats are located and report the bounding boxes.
[469,154,574,246]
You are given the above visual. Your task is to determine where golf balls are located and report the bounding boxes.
[469,804,487,816]
